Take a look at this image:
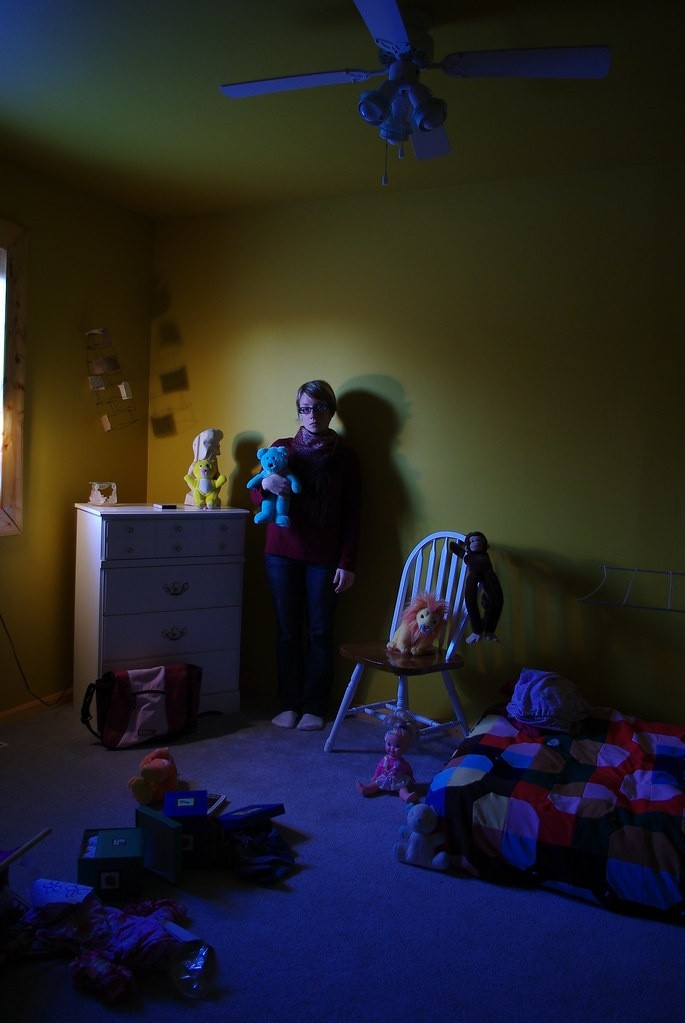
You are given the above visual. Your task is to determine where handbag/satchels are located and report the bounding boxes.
[81,662,203,748]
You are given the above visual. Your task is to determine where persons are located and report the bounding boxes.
[356,720,418,803]
[250,380,361,731]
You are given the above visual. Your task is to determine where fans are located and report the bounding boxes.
[219,0,613,98]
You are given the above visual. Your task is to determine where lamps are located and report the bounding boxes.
[358,83,448,146]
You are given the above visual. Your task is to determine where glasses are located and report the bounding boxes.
[298,405,330,414]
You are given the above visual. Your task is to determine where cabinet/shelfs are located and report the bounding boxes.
[73,502,249,737]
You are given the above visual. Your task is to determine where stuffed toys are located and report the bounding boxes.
[247,446,301,527]
[184,459,227,509]
[450,531,504,643]
[386,597,447,656]
[392,802,449,869]
[127,748,179,804]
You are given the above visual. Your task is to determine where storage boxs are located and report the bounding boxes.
[77,827,145,901]
[135,806,181,881]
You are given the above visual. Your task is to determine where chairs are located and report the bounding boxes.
[323,530,468,754]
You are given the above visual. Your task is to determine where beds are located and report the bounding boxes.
[424,702,685,914]
